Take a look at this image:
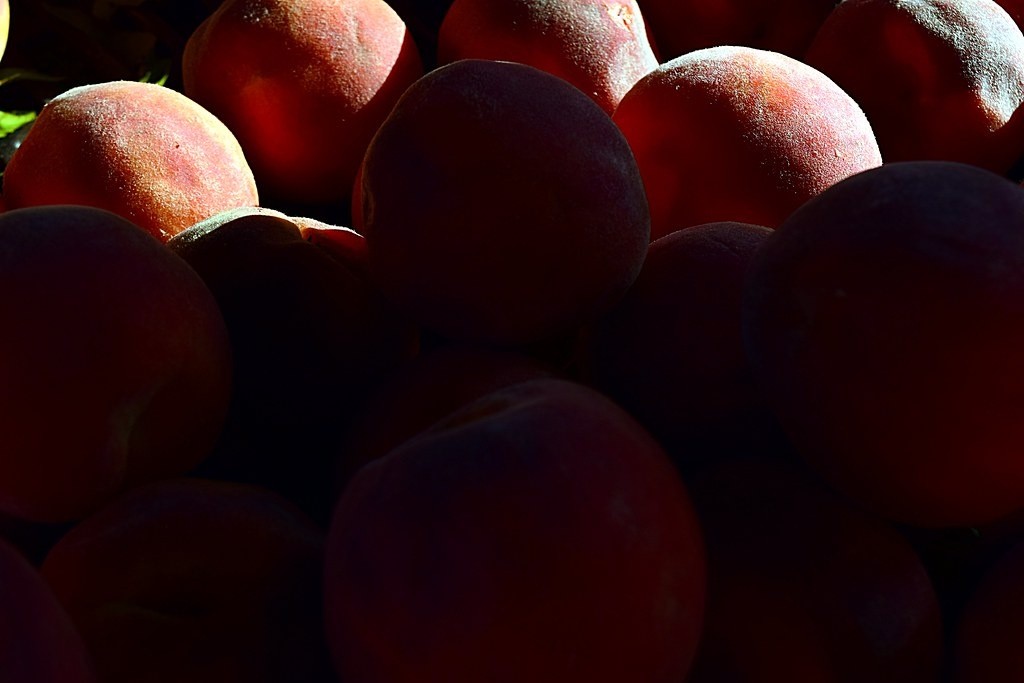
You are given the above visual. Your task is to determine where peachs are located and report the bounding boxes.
[0,0,1024,683]
